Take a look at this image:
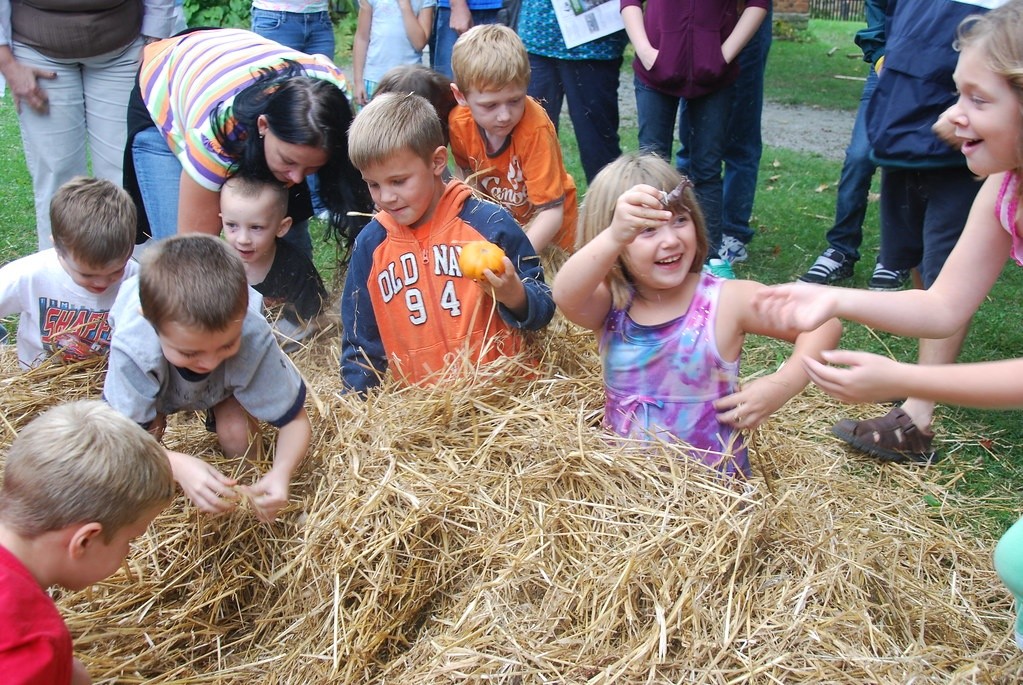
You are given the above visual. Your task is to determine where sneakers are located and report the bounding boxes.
[802,248,853,284]
[719,234,749,267]
[868,262,910,291]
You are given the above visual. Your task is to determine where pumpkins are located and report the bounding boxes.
[459,240,507,281]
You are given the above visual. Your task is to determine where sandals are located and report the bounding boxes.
[832,408,936,460]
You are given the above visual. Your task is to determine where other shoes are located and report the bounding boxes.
[270,311,330,355]
[701,256,736,280]
[319,209,342,222]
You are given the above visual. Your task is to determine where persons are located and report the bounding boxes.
[448,24,578,258]
[434,0,502,80]
[251,0,335,219]
[551,156,843,481]
[0,0,186,252]
[617,0,772,279]
[798,64,909,291]
[504,0,629,183]
[0,178,140,376]
[123,27,352,245]
[100,234,311,524]
[352,0,438,112]
[834,0,1012,463]
[217,175,328,351]
[749,0,1023,645]
[0,401,174,685]
[340,93,557,401]
